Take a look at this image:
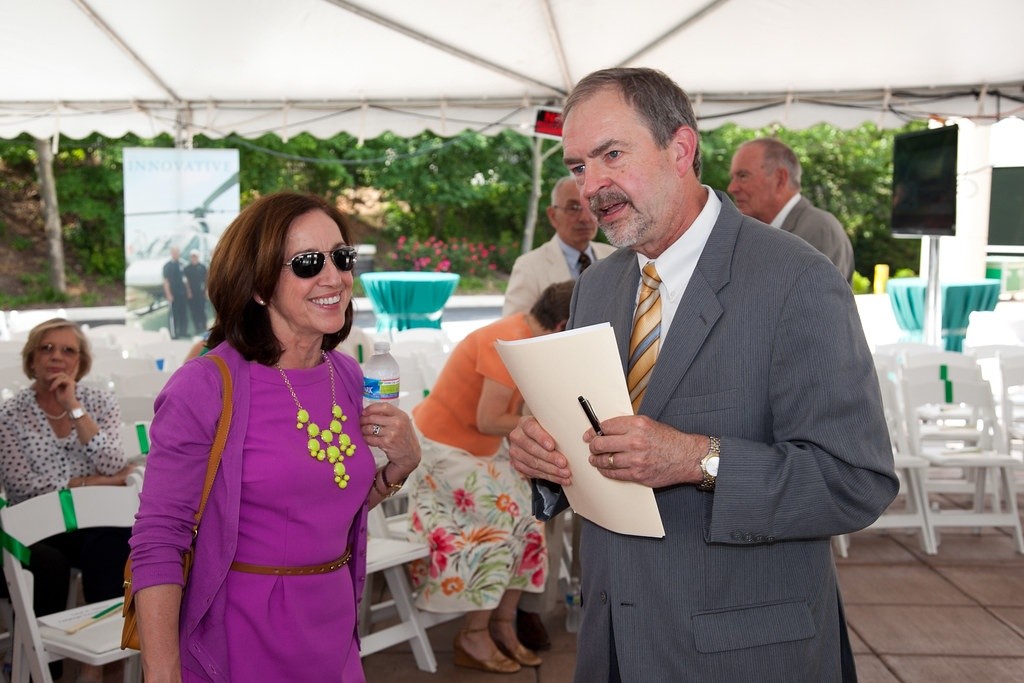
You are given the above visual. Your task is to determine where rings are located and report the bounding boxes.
[372,425,380,436]
[608,453,616,469]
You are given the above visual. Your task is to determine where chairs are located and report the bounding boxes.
[0,389,584,683]
[826,342,1024,554]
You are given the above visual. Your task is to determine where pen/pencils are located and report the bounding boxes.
[578,396,604,436]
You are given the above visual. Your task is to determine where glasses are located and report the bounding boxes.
[552,203,594,214]
[34,342,82,356]
[279,246,357,278]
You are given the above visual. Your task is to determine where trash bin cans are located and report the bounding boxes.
[351,245,376,273]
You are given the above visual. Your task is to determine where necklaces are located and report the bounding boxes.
[275,349,356,489]
[45,410,68,420]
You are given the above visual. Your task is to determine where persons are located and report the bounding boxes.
[503,174,627,321]
[130,191,423,683]
[404,280,579,674]
[0,318,137,683]
[163,246,210,342]
[509,66,900,683]
[726,137,858,280]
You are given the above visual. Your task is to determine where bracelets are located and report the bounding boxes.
[373,473,396,498]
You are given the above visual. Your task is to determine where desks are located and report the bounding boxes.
[359,270,461,336]
[886,276,1003,351]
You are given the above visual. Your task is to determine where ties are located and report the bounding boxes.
[579,252,591,274]
[627,262,662,416]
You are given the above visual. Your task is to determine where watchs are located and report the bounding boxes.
[70,405,87,418]
[381,461,408,491]
[700,436,721,488]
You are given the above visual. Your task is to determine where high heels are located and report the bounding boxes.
[490,632,543,667]
[454,627,522,674]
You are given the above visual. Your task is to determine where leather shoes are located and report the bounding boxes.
[516,606,551,651]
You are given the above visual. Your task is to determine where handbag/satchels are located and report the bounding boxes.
[119,354,234,650]
